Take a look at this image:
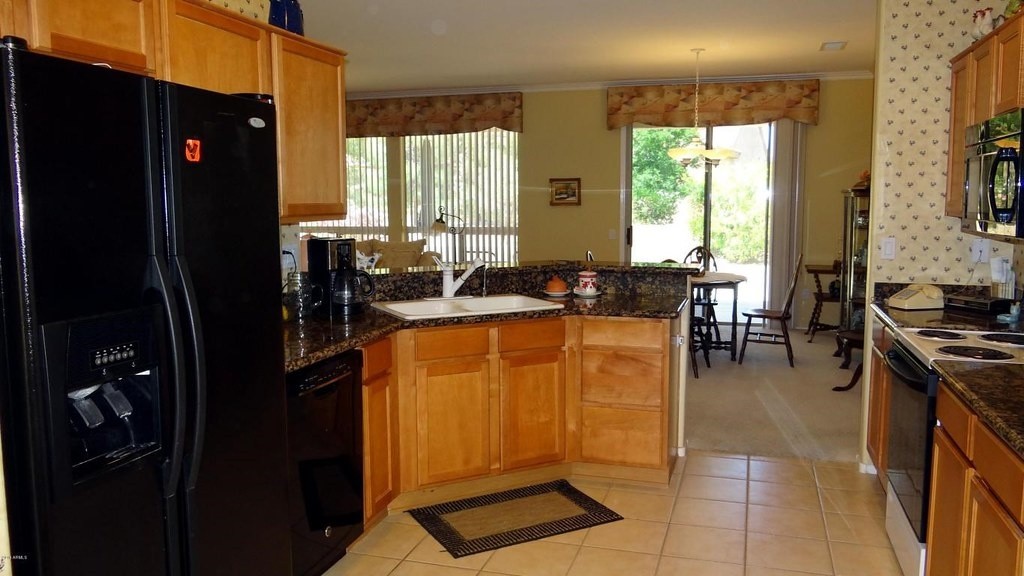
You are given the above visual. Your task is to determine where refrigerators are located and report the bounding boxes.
[0,36,293,576]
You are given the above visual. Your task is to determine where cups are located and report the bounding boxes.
[287,272,323,318]
[577,271,597,293]
[543,290,570,296]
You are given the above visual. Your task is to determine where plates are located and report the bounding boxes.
[573,290,601,298]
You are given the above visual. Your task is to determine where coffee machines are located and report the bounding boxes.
[307,237,362,315]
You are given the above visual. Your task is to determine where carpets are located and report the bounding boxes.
[408,477,623,558]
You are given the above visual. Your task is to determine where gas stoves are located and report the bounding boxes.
[894,327,1024,369]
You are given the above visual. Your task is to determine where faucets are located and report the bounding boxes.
[432,255,444,270]
[443,258,486,300]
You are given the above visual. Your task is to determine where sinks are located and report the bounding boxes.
[371,299,475,321]
[454,294,565,315]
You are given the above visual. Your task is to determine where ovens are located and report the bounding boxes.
[285,349,365,576]
[884,339,939,543]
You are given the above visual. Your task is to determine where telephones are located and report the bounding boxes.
[889,285,944,309]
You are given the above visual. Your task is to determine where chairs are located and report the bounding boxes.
[662,247,803,392]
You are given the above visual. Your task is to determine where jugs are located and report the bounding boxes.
[329,268,374,315]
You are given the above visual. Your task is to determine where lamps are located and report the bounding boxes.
[668,49,741,169]
[432,206,465,264]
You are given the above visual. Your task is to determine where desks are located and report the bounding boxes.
[805,265,847,344]
[689,272,747,361]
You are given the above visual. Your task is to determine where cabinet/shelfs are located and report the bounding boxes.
[868,0,1024,576]
[832,174,871,370]
[348,316,675,550]
[0,0,349,225]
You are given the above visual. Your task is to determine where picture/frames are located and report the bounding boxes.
[549,178,581,206]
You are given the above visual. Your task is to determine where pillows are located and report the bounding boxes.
[357,240,426,268]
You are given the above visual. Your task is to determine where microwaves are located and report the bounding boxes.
[961,107,1024,245]
